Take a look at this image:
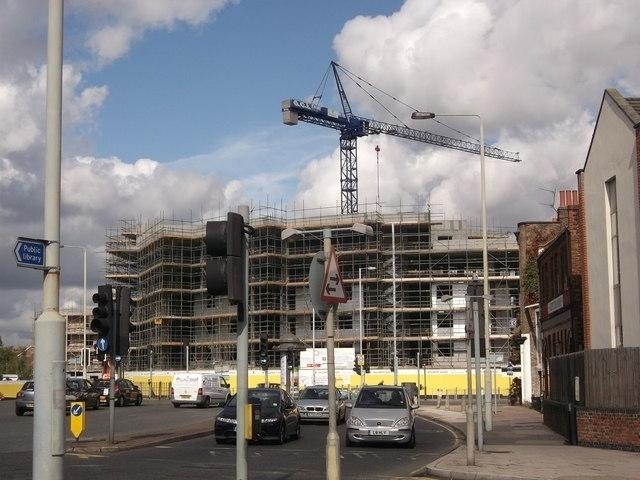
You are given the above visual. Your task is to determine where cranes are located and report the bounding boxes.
[280,61,522,216]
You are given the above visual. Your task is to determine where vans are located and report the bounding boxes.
[171,371,232,408]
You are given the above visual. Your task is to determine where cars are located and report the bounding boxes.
[215,372,420,446]
[16,377,143,416]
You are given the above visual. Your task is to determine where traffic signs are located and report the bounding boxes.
[14,239,46,267]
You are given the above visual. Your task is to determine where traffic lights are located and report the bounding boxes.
[91,285,137,361]
[258,333,271,371]
[206,212,244,303]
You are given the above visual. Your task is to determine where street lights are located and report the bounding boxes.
[280,223,377,480]
[411,112,493,466]
